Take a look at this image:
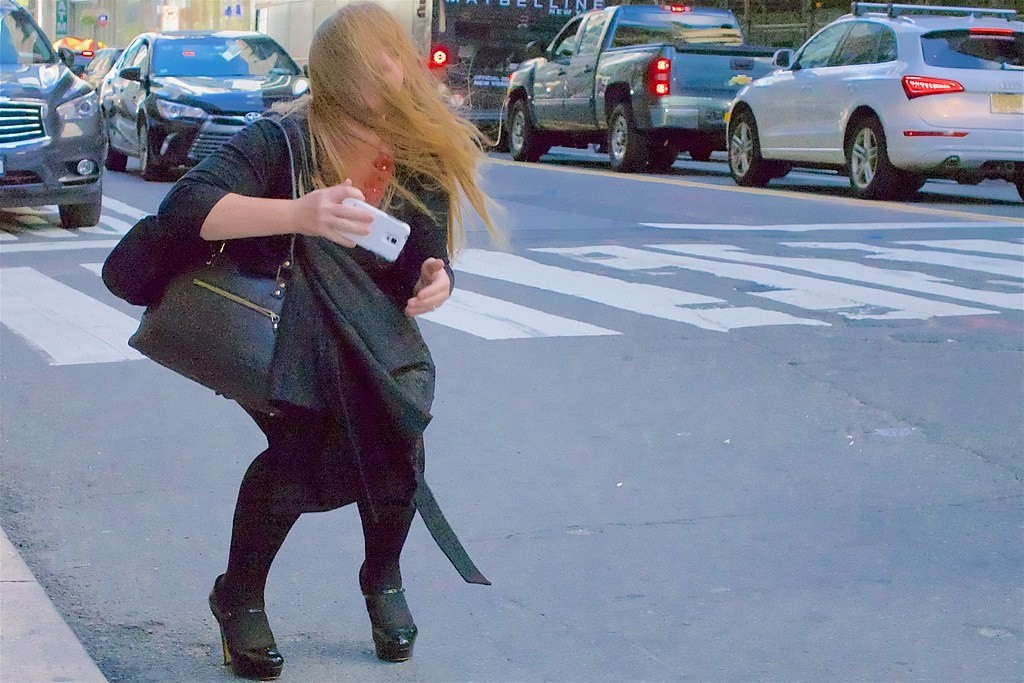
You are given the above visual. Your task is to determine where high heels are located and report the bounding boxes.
[360,560,418,660]
[209,574,284,680]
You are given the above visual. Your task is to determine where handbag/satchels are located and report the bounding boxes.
[128,110,311,418]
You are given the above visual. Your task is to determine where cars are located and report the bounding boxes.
[100,30,309,181]
[1,0,104,226]
[725,3,1024,199]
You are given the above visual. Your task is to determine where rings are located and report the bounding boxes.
[430,304,437,313]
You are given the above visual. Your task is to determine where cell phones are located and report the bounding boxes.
[333,198,411,263]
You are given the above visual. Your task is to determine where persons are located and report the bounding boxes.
[162,1,490,678]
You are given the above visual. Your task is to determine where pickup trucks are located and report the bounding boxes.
[505,3,799,172]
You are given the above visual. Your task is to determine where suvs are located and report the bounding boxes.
[81,49,125,91]
[70,52,94,76]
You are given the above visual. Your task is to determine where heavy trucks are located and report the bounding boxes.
[257,0,619,150]
